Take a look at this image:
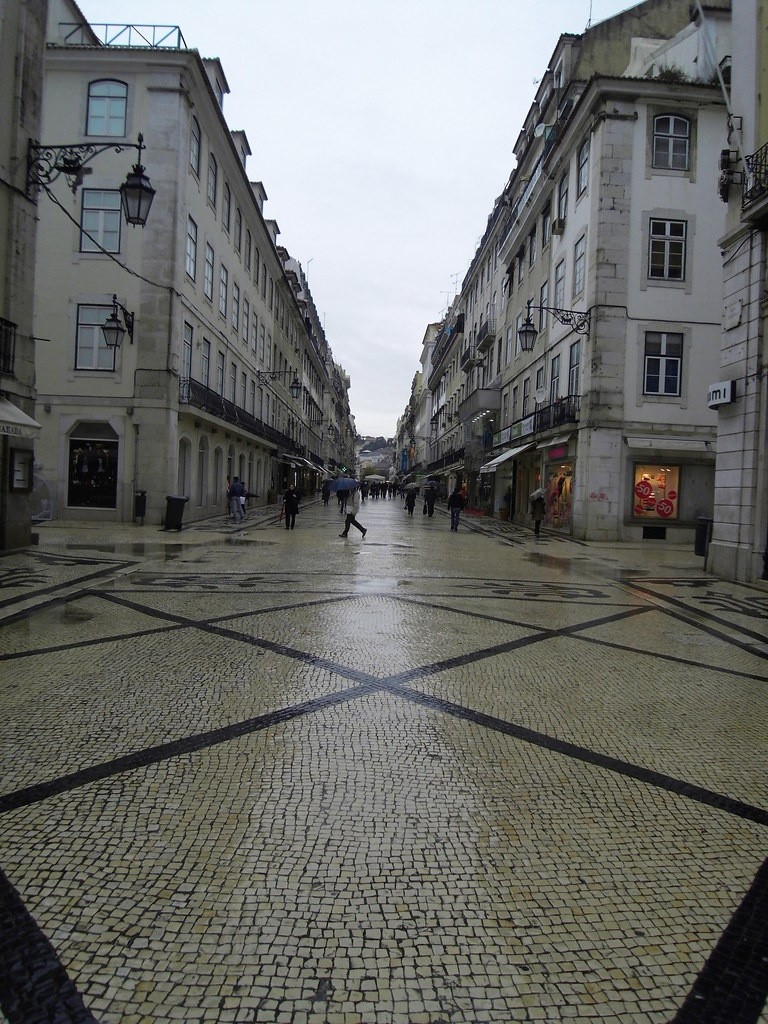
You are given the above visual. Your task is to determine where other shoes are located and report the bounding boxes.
[361,528,367,537]
[285,527,289,530]
[338,534,348,538]
[291,526,294,529]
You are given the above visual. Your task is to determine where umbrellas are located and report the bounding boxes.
[529,487,545,503]
[246,492,260,498]
[427,481,439,486]
[404,482,422,490]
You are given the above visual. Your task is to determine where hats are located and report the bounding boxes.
[240,481,245,485]
[232,477,239,481]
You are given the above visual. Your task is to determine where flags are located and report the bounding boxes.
[643,16,719,84]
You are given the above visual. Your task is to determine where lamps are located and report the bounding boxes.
[310,418,334,435]
[257,368,302,399]
[666,467,671,472]
[25,132,156,230]
[409,433,431,448]
[127,406,134,415]
[44,404,52,413]
[660,467,665,471]
[704,440,712,450]
[100,293,135,349]
[517,296,591,353]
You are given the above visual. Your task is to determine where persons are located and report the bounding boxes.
[321,481,330,506]
[338,487,367,537]
[424,485,440,517]
[532,496,546,538]
[226,475,252,524]
[283,483,301,530]
[357,478,406,501]
[336,489,350,514]
[447,487,464,532]
[329,478,362,490]
[405,489,416,516]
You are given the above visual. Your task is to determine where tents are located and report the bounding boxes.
[365,474,386,484]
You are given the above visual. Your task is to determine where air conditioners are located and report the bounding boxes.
[551,218,564,235]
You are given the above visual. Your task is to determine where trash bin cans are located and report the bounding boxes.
[694,516,714,557]
[134,489,147,516]
[164,495,188,532]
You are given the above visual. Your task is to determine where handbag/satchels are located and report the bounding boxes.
[404,505,407,510]
[422,505,427,515]
[542,506,546,515]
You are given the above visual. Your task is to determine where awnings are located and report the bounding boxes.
[423,465,464,479]
[0,394,42,440]
[283,454,342,476]
[536,432,571,448]
[479,441,538,473]
[624,438,711,452]
